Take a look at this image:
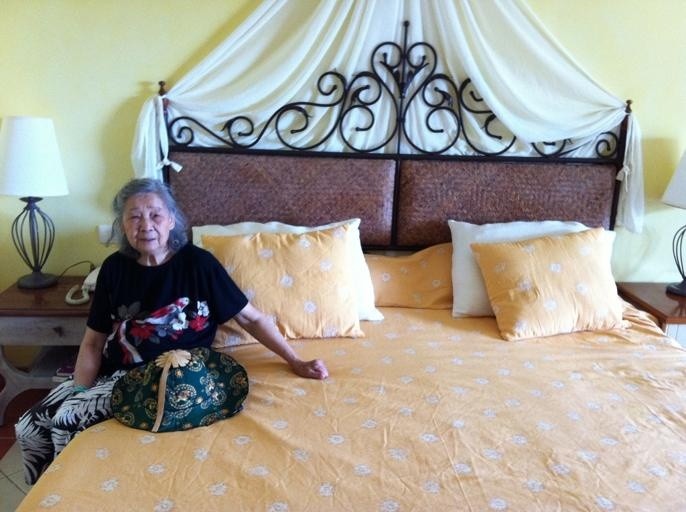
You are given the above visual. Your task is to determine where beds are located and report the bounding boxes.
[15,21,686,512]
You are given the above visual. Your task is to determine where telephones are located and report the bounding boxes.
[81,266,101,292]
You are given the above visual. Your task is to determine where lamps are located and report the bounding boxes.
[660,150,686,298]
[0,117,69,289]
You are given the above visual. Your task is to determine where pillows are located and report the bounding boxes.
[191,217,385,348]
[468,225,623,342]
[364,242,452,309]
[448,219,592,318]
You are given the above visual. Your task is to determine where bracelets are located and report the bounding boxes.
[72,385,87,393]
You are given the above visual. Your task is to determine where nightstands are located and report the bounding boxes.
[0,276,93,426]
[617,283,686,345]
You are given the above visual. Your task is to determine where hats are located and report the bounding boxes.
[110,347,249,433]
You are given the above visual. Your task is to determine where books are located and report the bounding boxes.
[55,357,74,376]
[51,374,69,383]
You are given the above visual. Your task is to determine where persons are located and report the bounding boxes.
[13,177,330,488]
